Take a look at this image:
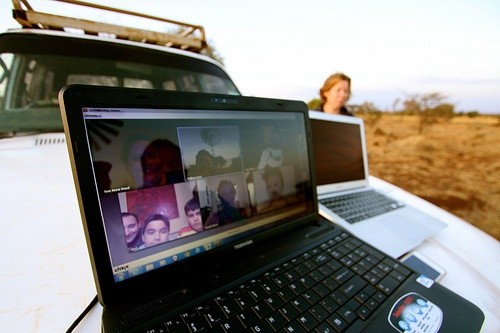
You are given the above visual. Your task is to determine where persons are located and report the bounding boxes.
[215,179,248,223]
[123,133,162,187]
[179,199,202,238]
[261,168,297,211]
[122,212,144,251]
[196,149,218,177]
[141,214,177,248]
[257,128,293,169]
[317,73,353,115]
[140,138,183,189]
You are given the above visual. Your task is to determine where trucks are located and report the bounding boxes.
[0,1,243,333]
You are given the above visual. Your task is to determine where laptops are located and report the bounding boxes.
[308,110,450,260]
[58,83,485,333]
